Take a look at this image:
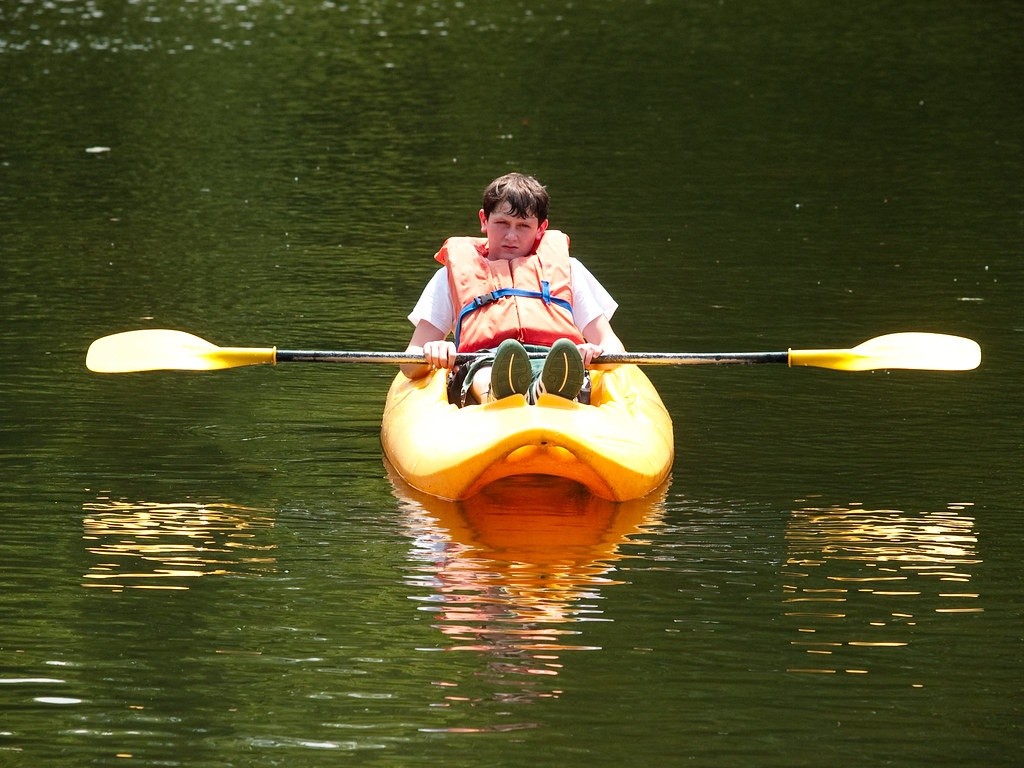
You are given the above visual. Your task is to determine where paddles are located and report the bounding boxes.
[85,328,982,375]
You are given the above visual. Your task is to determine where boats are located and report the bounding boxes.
[381,366,676,502]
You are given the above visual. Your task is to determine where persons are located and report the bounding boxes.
[400,173,625,405]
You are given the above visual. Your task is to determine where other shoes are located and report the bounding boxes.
[486,338,532,403]
[525,337,585,405]
